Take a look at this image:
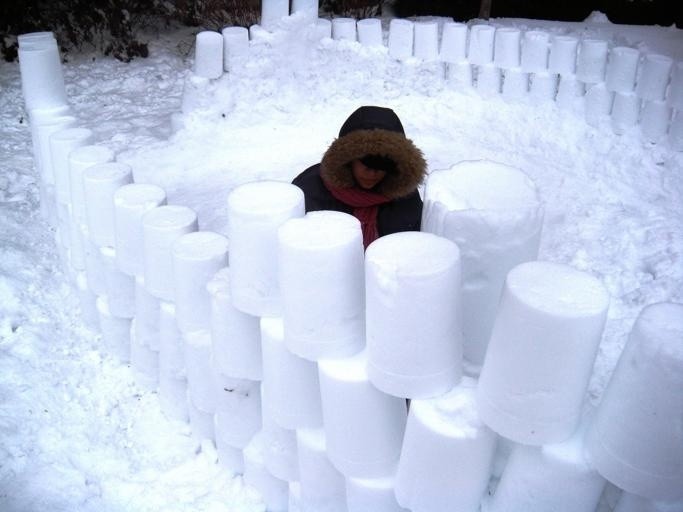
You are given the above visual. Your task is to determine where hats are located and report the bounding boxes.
[361,154,394,173]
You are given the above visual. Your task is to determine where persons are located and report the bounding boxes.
[291,105,423,250]
